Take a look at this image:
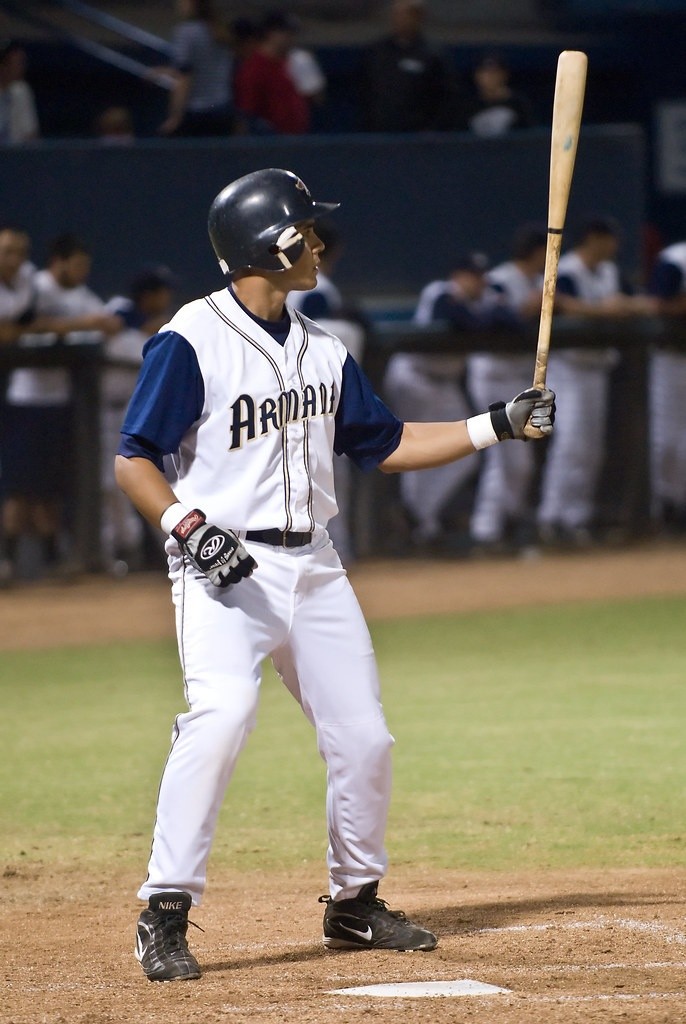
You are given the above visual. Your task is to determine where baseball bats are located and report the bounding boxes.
[523,48,587,440]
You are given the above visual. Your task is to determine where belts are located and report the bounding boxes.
[246,527,311,549]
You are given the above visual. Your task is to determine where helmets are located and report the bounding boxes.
[207,168,340,275]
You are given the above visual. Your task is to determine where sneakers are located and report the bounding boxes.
[318,879,439,950]
[133,891,207,981]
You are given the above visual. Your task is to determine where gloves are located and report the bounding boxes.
[171,509,258,587]
[488,387,556,442]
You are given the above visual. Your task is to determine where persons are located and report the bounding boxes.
[362,0,454,132]
[463,45,535,142]
[113,168,556,982]
[0,0,322,139]
[0,223,374,588]
[648,240,686,503]
[399,232,657,554]
